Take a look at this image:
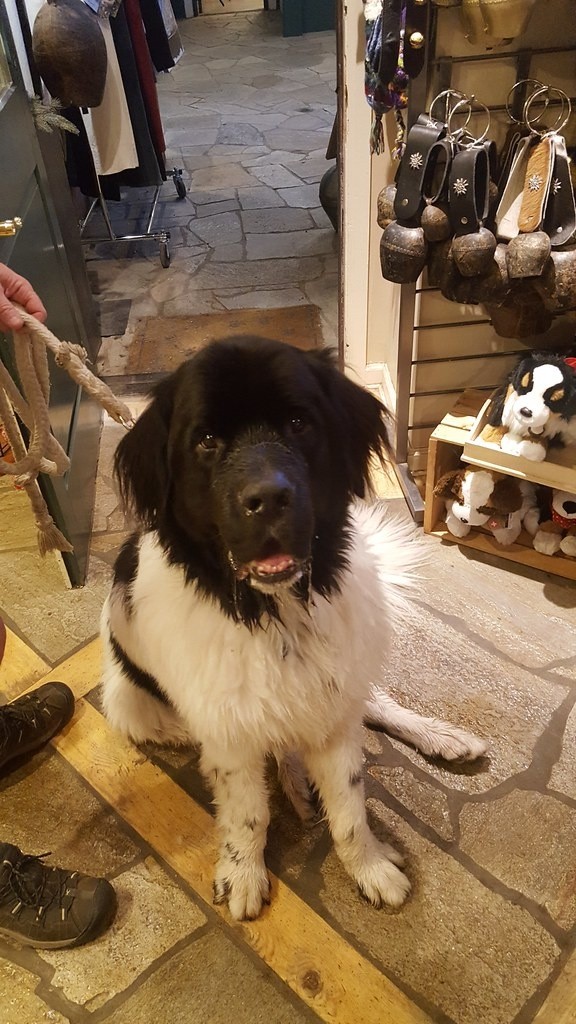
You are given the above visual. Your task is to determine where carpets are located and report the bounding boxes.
[126,303,327,374]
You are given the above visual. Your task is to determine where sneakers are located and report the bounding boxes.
[0,681,75,781]
[0,840,117,950]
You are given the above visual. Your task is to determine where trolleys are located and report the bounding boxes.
[76,107,187,270]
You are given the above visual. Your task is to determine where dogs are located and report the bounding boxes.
[102,335,490,924]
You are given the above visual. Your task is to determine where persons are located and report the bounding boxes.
[0,257,117,951]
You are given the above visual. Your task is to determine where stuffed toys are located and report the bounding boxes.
[433,466,576,558]
[480,354,576,463]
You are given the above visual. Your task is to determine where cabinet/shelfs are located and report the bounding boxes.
[423,386,576,582]
